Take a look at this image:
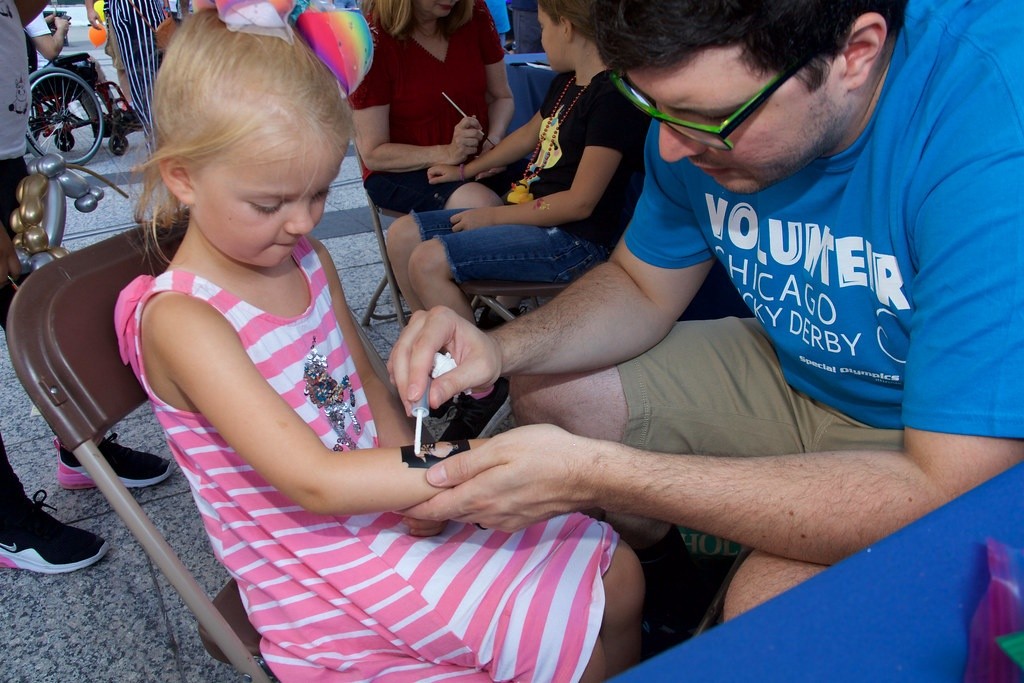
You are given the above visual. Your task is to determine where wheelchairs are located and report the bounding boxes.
[23,30,145,166]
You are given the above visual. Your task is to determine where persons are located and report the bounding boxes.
[386,0,652,441]
[347,0,514,214]
[387,0,1024,624]
[115,0,646,683]
[23,11,122,133]
[0,0,194,574]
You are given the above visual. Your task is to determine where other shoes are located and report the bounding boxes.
[478,304,529,331]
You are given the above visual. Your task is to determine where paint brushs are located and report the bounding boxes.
[442,92,495,146]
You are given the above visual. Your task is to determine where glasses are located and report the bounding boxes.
[610,51,839,146]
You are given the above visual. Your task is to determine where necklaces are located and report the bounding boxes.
[508,74,590,204]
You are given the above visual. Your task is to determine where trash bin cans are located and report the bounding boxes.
[43,11,69,46]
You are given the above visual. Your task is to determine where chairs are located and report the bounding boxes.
[7,209,436,682]
[349,114,588,335]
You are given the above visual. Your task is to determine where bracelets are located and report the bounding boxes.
[460,164,464,180]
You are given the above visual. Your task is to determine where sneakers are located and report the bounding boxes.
[433,377,512,443]
[0,486,110,575]
[52,431,174,490]
